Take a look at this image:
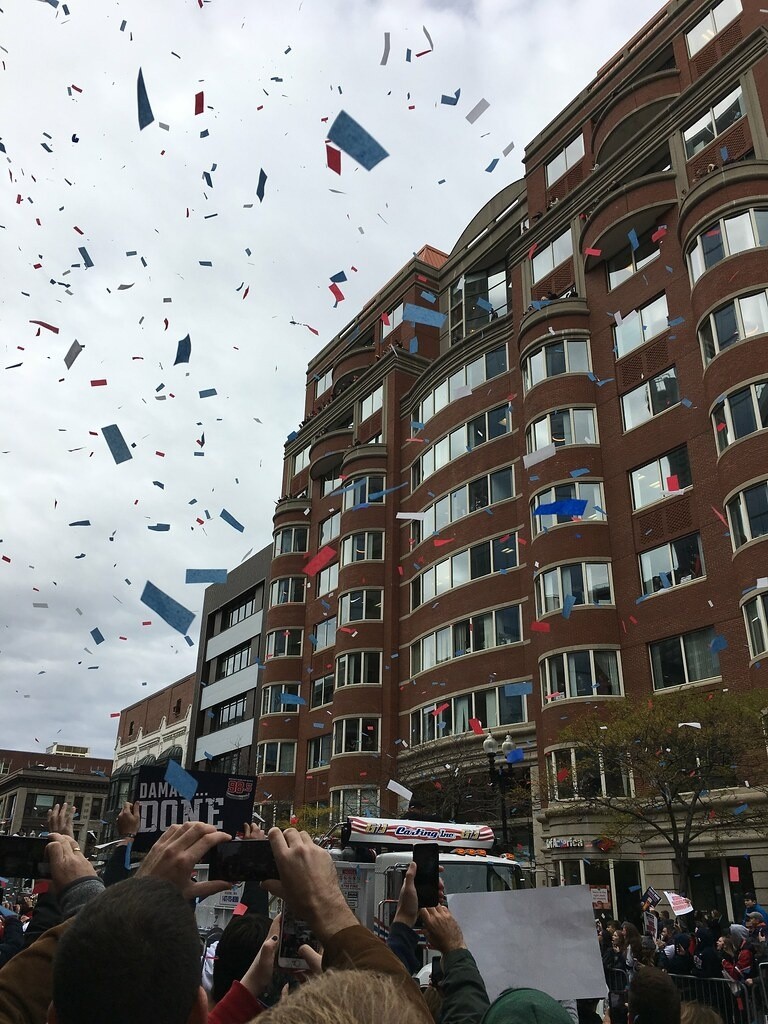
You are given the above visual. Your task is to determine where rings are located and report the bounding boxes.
[73,847,82,853]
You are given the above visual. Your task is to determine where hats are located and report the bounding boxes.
[744,893,756,900]
[206,927,224,947]
[746,911,763,920]
[479,988,575,1024]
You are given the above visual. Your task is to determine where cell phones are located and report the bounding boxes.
[279,901,320,969]
[413,843,440,909]
[208,839,280,881]
[0,835,55,881]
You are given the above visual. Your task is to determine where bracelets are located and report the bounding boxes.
[119,832,136,841]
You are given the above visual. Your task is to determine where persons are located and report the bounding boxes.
[0,801,768,1024]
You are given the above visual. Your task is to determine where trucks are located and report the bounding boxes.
[189,814,537,995]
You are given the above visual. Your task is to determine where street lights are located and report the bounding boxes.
[483,726,519,853]
